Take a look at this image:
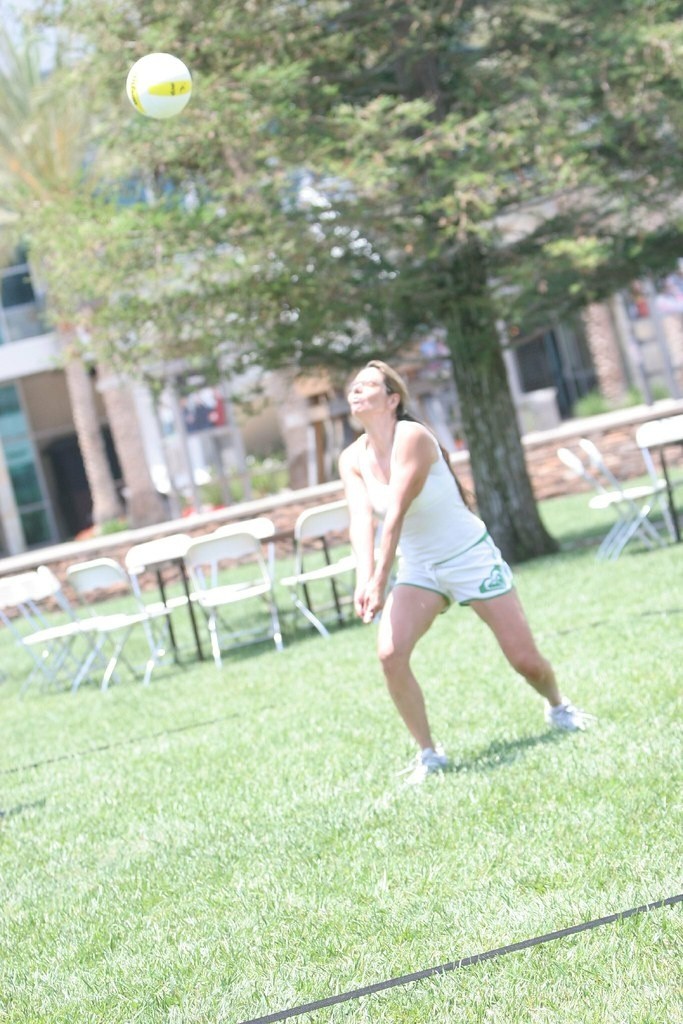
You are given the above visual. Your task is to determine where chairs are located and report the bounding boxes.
[0,498,398,696]
[557,439,667,562]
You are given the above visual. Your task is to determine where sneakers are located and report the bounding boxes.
[544,696,597,732]
[396,743,447,784]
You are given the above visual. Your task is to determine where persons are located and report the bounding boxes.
[339,360,599,784]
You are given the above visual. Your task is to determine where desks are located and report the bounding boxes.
[635,412,683,544]
[142,517,345,664]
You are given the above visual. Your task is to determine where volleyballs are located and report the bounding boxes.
[126,51,192,120]
